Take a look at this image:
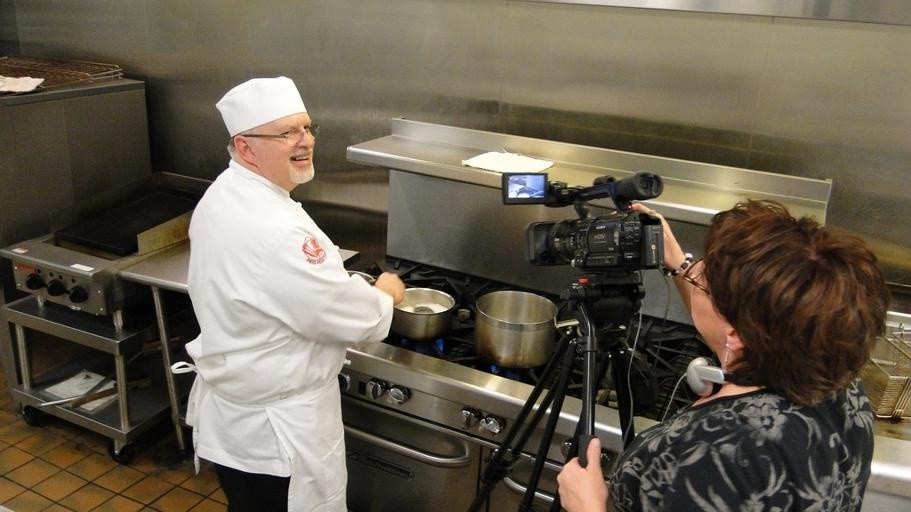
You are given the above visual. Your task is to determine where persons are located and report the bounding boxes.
[178,74,408,511]
[552,196,891,512]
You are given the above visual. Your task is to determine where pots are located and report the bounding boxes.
[474,289,560,370]
[391,287,456,340]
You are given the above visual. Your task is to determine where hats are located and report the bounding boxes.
[215,76,307,138]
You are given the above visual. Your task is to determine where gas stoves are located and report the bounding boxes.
[339,255,723,476]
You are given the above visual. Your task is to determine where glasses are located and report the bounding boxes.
[233,124,320,152]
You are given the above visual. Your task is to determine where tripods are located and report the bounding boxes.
[469,332,636,511]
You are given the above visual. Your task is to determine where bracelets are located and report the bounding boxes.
[661,250,694,279]
